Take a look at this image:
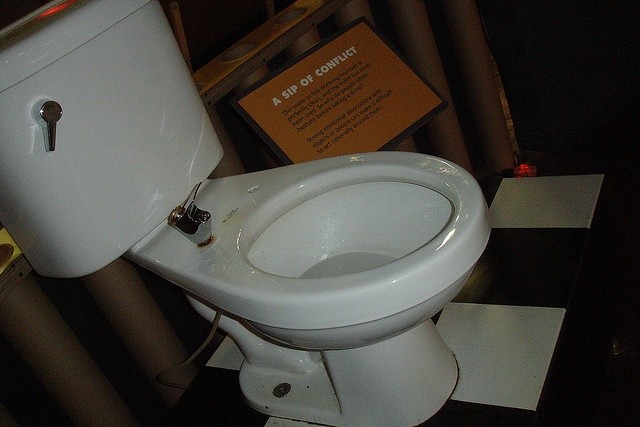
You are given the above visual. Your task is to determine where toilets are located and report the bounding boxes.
[0,1,492,426]
